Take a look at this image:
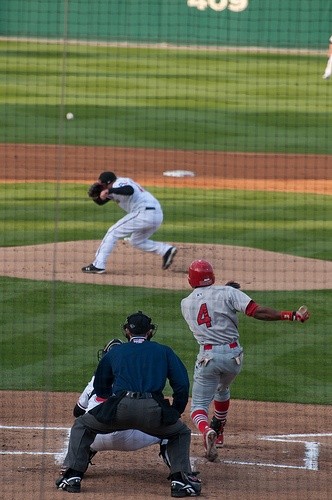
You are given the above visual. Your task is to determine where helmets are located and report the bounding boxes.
[188,259,215,288]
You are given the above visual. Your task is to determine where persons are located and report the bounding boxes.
[181,260,310,462]
[60,339,202,486]
[55,311,202,498]
[81,171,177,274]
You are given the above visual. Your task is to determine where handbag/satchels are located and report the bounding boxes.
[88,395,120,424]
[152,391,182,427]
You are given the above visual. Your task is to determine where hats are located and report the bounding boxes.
[125,311,151,334]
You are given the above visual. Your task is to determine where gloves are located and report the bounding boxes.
[293,311,309,322]
[225,282,240,289]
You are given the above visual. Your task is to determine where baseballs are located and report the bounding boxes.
[65,112,74,120]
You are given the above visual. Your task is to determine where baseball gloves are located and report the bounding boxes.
[87,182,104,204]
[224,280,241,290]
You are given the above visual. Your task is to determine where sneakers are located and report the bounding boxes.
[203,429,219,462]
[171,481,201,497]
[56,476,80,493]
[162,246,177,270]
[82,263,106,274]
[217,433,224,448]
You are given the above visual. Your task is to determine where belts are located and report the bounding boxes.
[204,341,238,350]
[126,390,151,399]
[145,207,155,210]
